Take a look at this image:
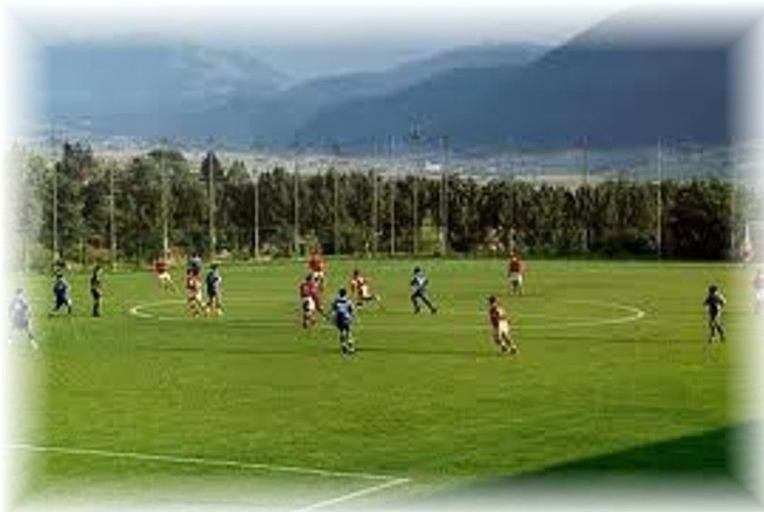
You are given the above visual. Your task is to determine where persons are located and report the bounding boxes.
[153,254,179,294]
[184,250,229,318]
[48,273,74,315]
[749,264,763,313]
[409,266,439,316]
[90,265,105,318]
[505,251,529,298]
[487,295,518,357]
[701,283,729,344]
[298,246,382,359]
[10,287,39,353]
